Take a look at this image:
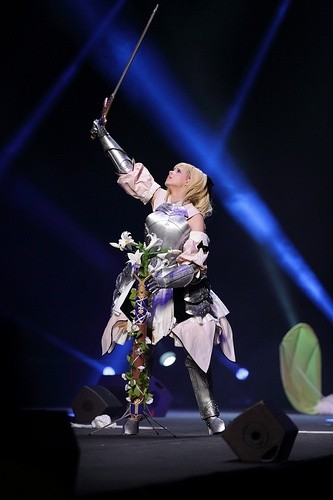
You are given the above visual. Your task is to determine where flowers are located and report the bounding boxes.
[110,231,182,277]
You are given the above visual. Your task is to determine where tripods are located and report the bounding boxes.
[89,404,177,437]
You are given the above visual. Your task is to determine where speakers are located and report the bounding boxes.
[72,385,122,425]
[0,409,79,500]
[222,399,299,463]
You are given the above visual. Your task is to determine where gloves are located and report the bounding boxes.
[147,277,166,294]
[91,120,105,139]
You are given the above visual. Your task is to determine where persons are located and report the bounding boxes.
[89,120,236,435]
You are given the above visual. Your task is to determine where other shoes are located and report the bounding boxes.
[205,417,226,436]
[123,419,139,436]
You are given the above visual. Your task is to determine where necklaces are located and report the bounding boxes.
[165,189,182,204]
